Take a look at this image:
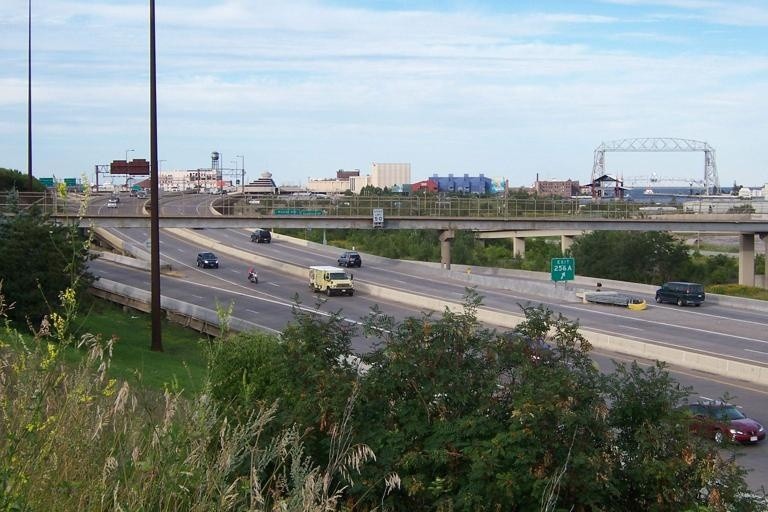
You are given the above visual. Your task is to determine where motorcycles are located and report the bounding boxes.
[248,270,258,284]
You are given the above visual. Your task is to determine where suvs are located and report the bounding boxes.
[338,252,361,267]
[197,252,219,269]
[250,230,270,244]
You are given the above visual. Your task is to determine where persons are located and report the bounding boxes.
[247,265,255,279]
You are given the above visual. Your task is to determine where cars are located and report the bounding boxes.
[685,402,765,444]
[204,187,227,195]
[130,190,146,199]
[107,196,119,208]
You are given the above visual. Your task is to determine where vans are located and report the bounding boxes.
[655,283,704,308]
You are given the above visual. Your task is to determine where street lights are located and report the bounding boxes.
[126,149,135,192]
[230,155,245,195]
[158,160,167,195]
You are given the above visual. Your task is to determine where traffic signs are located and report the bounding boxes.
[64,178,76,186]
[40,178,52,188]
[549,257,575,281]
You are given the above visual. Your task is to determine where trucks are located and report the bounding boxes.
[308,266,354,297]
